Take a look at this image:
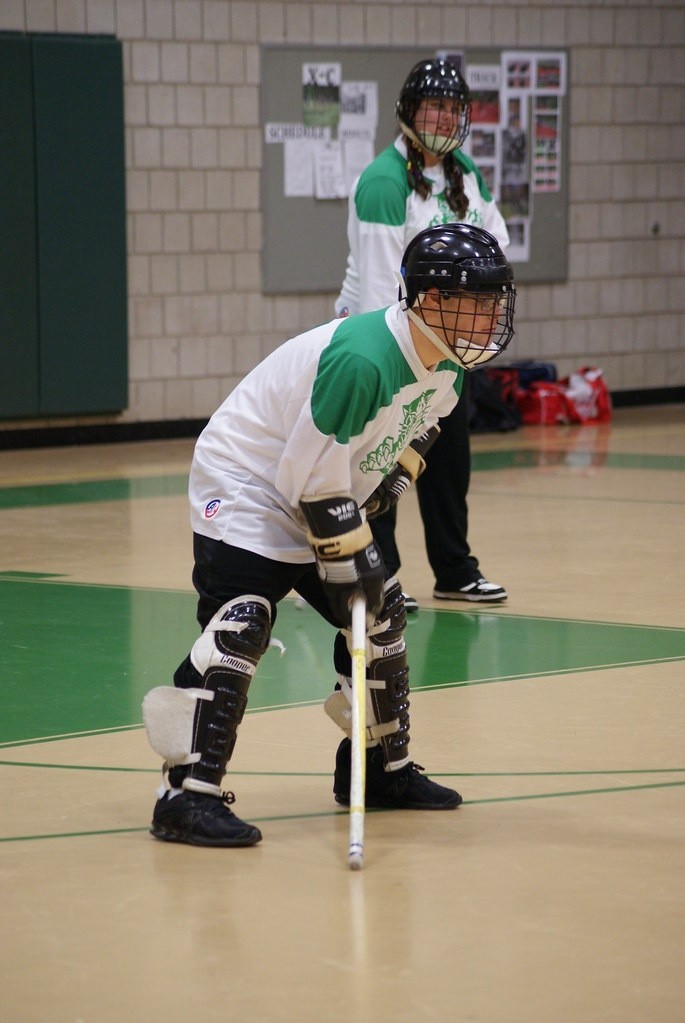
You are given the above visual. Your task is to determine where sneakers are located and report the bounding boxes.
[148,779,263,846]
[432,574,507,602]
[334,739,464,811]
[409,596,420,612]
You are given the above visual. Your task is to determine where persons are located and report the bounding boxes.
[333,58,510,611]
[141,224,515,846]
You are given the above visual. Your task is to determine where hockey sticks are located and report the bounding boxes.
[346,589,368,871]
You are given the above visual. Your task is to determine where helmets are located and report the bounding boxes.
[396,57,468,156]
[401,222,516,371]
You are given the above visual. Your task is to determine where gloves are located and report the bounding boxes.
[297,491,386,632]
[359,445,427,519]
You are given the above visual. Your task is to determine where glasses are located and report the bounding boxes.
[472,296,506,312]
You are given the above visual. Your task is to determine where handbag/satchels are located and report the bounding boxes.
[522,381,569,425]
[557,364,612,425]
